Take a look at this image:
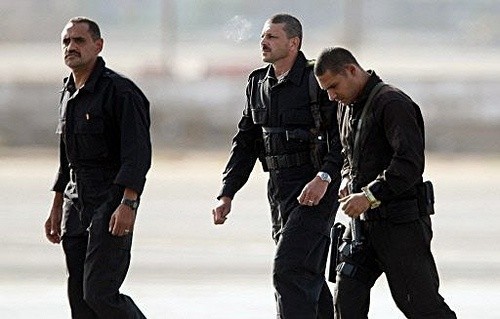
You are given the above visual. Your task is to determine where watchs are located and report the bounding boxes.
[361,188,381,210]
[121,199,138,210]
[316,172,332,184]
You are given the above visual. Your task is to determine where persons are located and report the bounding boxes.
[212,14,344,319]
[313,48,457,319]
[44,17,152,319]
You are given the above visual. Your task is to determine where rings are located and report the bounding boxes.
[125,229,130,232]
[308,200,313,203]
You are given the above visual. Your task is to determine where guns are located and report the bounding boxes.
[347,176,363,243]
